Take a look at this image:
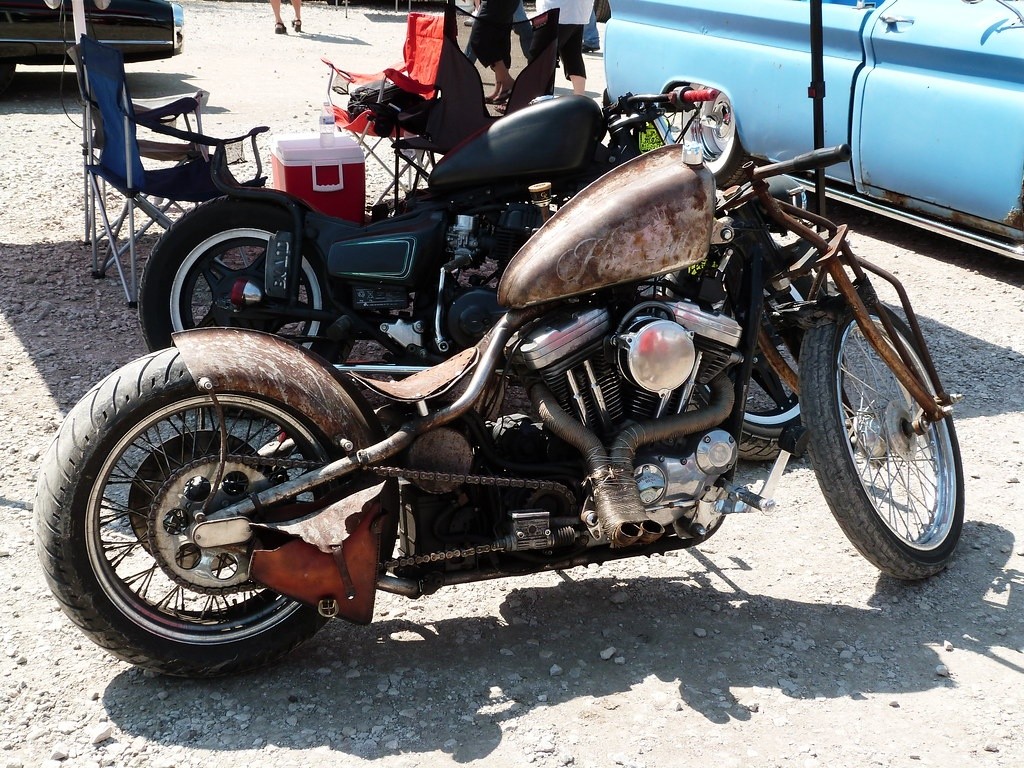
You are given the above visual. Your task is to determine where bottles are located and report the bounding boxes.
[319,102,337,148]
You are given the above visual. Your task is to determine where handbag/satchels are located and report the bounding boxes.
[593,0,612,24]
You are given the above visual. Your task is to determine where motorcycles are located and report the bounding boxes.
[135,78,807,371]
[30,138,965,681]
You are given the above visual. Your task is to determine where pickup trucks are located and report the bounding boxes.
[603,0,1024,260]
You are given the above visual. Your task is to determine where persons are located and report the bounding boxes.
[268,0,302,35]
[582,0,600,53]
[534,0,587,96]
[464,0,555,115]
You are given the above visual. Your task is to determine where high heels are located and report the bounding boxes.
[275,22,286,34]
[291,19,302,32]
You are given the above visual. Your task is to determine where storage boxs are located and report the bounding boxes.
[268,134,365,224]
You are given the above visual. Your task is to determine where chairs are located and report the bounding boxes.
[80,34,270,309]
[390,4,560,209]
[66,43,249,248]
[319,11,444,205]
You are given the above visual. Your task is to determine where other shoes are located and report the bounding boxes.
[494,104,507,114]
[484,95,496,103]
[493,86,515,105]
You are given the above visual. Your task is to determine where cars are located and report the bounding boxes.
[0,0,182,100]
